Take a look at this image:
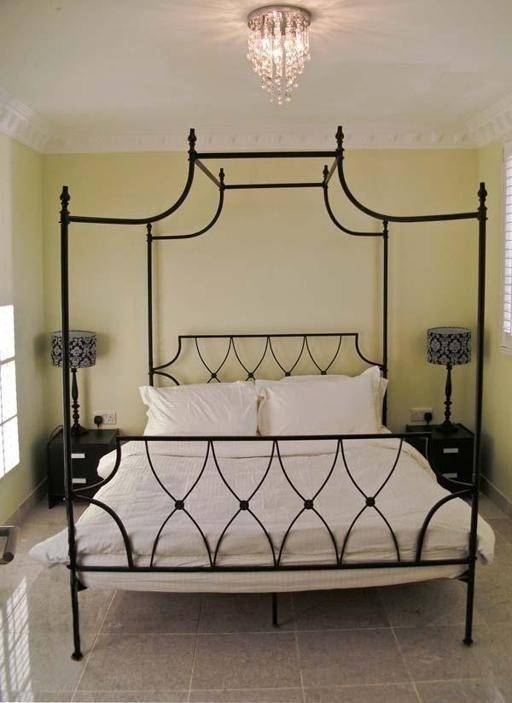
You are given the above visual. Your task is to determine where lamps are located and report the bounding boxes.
[426,326,472,431]
[245,5,314,107]
[48,329,96,437]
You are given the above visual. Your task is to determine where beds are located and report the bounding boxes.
[61,332,485,659]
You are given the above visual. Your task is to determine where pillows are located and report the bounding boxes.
[139,362,392,435]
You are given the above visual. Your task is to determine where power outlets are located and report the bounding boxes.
[410,407,432,422]
[93,410,116,424]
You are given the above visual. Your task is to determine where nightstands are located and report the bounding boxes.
[46,428,119,509]
[405,423,475,500]
